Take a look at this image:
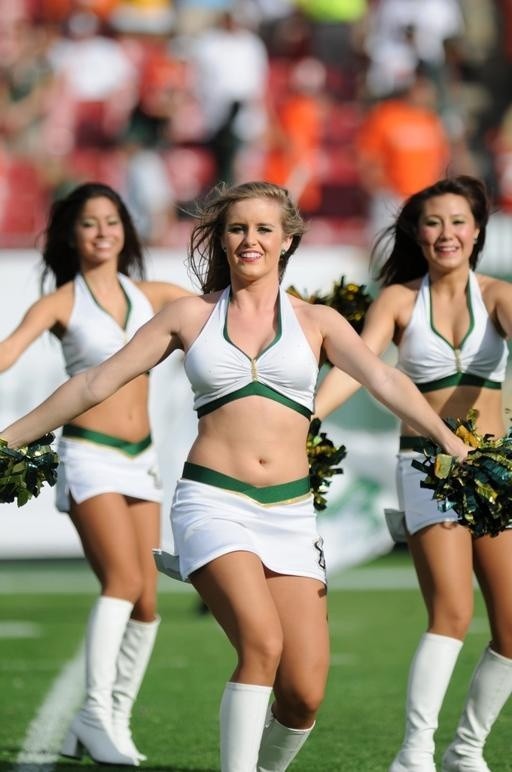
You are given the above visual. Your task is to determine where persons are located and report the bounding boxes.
[0,176,475,772]
[0,179,200,766]
[309,175,512,772]
[0,0,511,247]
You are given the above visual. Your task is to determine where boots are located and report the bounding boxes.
[441,643,511,772]
[257,702,315,770]
[220,682,271,772]
[61,594,162,765]
[388,633,464,772]
